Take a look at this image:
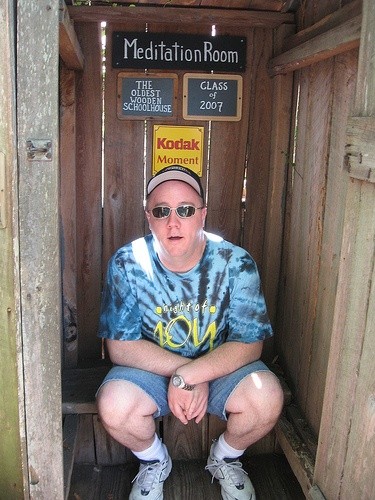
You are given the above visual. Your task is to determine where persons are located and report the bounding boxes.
[95,164,284,500]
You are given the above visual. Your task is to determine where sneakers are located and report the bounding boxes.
[128,438,172,500]
[204,438,256,500]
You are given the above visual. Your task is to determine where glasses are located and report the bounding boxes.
[147,205,205,220]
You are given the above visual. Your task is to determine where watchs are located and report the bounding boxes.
[171,369,195,392]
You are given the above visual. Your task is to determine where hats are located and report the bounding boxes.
[145,164,204,202]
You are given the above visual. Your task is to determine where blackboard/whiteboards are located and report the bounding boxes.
[182,72,243,121]
[116,71,179,121]
